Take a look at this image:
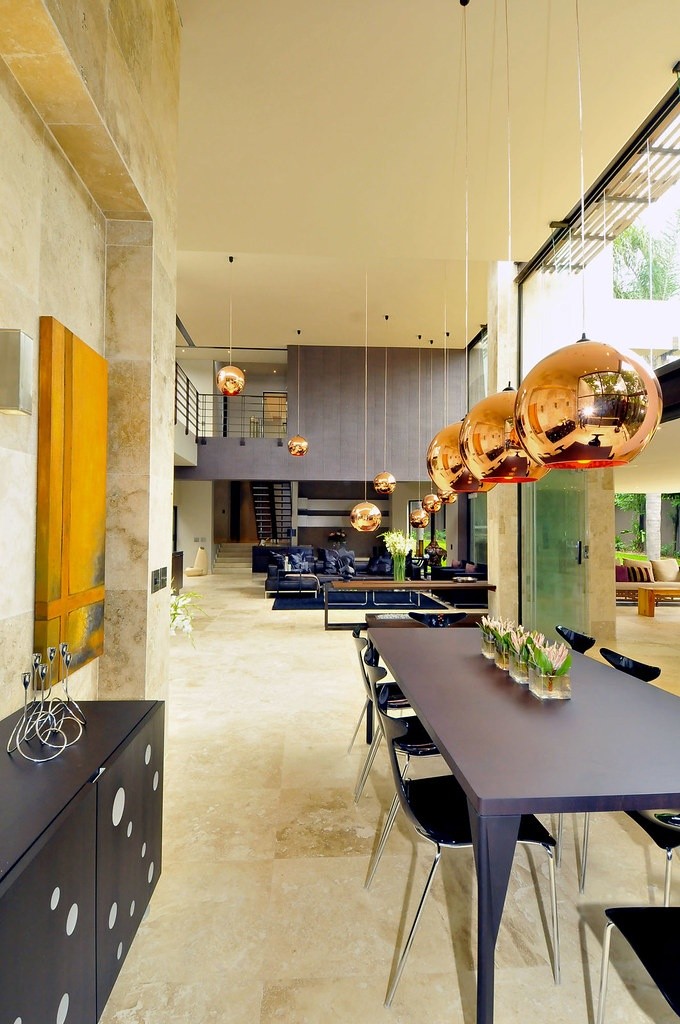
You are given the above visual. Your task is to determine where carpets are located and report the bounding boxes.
[272,593,448,610]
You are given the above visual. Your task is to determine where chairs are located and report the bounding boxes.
[346,611,680,1024]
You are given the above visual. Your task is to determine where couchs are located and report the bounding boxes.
[265,548,488,602]
[614,558,680,606]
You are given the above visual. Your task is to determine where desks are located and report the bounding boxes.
[637,585,680,616]
[367,627,680,1024]
[323,580,496,631]
[276,568,303,593]
[252,544,313,573]
[323,577,413,604]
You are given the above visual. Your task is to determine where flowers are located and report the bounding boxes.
[377,528,418,556]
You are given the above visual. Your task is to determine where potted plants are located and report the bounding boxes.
[476,614,571,701]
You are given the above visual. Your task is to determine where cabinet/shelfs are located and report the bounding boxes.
[0,698,166,1024]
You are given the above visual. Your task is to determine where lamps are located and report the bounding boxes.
[351,0,663,531]
[288,329,309,456]
[217,255,245,395]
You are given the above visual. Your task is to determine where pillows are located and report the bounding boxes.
[616,557,680,582]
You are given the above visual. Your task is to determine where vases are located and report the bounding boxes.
[394,554,406,582]
